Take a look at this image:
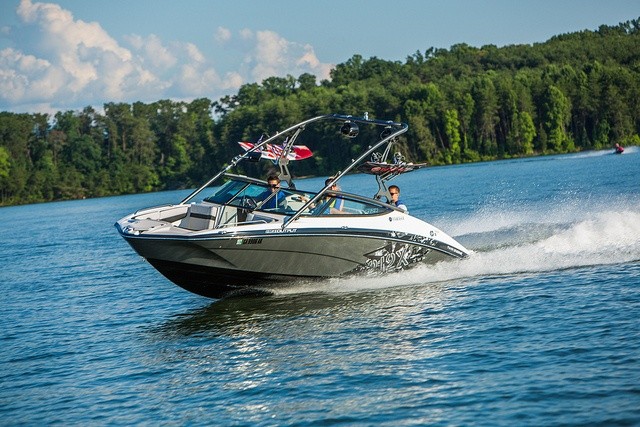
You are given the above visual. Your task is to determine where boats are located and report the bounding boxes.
[114,114,472,299]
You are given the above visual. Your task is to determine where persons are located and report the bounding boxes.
[615,142,624,153]
[308,197,352,214]
[256,175,287,208]
[322,178,345,211]
[381,184,408,213]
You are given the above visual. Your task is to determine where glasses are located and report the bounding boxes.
[269,183,278,186]
[330,184,336,188]
[390,193,397,196]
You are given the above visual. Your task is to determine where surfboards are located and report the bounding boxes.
[359,161,429,174]
[237,142,313,160]
